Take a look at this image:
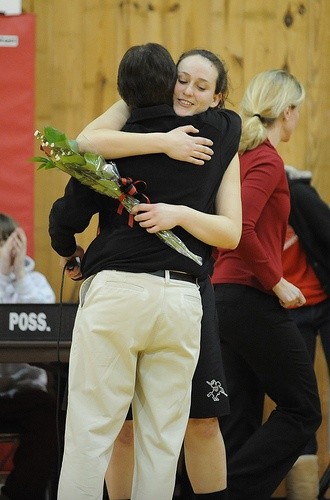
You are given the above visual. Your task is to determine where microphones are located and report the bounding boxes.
[67,256,83,281]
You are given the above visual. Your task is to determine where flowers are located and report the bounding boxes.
[34,127,203,268]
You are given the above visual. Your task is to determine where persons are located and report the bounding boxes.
[48,43,242,500]
[208,63,324,500]
[0,212,57,304]
[72,48,245,500]
